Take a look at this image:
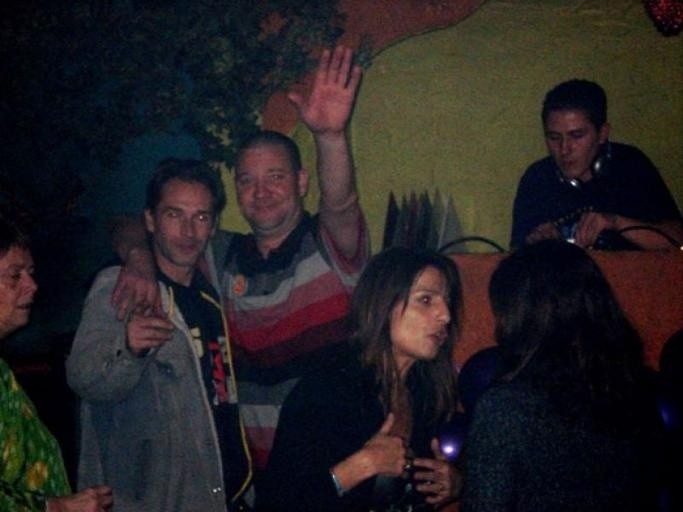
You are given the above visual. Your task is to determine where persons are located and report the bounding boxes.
[435,242,683,511]
[512,80,683,254]
[0,229,112,512]
[66,157,251,512]
[112,44,373,512]
[242,246,463,512]
